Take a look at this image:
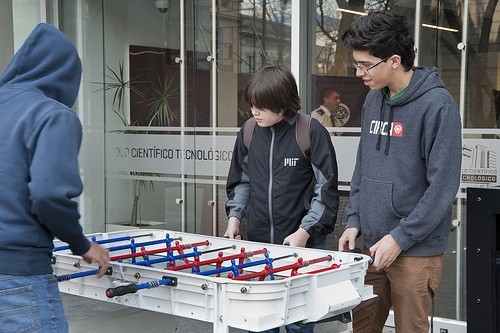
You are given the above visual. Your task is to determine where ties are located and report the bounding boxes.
[331,115,338,136]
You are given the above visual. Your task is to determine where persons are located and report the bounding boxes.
[0,23,110,333]
[338,11,461,333]
[311,87,343,136]
[226,64,340,333]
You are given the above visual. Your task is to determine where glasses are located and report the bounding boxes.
[352,55,391,75]
